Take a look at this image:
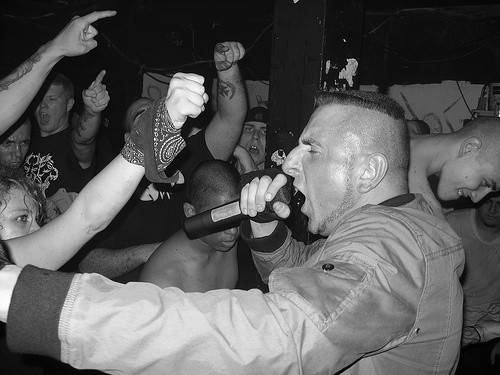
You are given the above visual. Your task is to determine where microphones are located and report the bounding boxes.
[183,186,291,239]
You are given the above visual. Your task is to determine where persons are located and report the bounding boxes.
[0,11,500,375]
[0,88,465,375]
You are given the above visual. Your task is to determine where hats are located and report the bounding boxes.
[244,106,269,125]
